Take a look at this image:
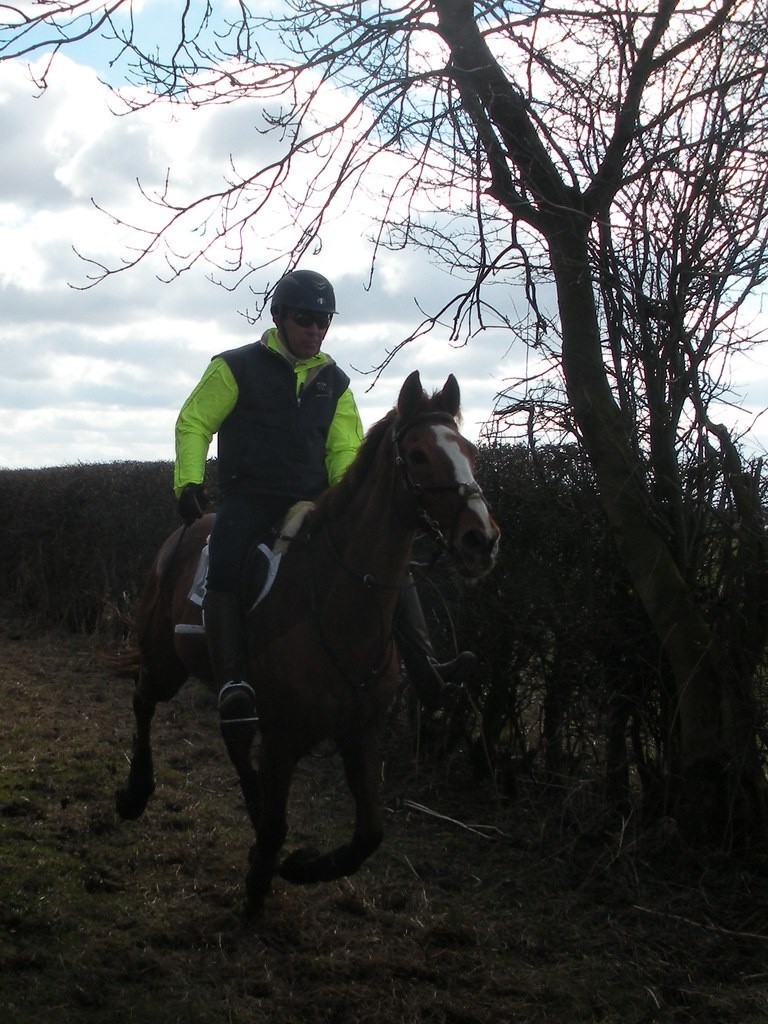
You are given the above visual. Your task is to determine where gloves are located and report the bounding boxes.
[178,483,209,524]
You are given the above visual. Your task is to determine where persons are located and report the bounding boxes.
[176,271,476,737]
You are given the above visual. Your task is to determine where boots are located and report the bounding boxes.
[202,590,256,743]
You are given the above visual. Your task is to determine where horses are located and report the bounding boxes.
[114,372,503,929]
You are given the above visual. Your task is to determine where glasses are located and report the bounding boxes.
[282,312,330,330]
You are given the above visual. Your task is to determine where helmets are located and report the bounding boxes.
[270,271,339,314]
